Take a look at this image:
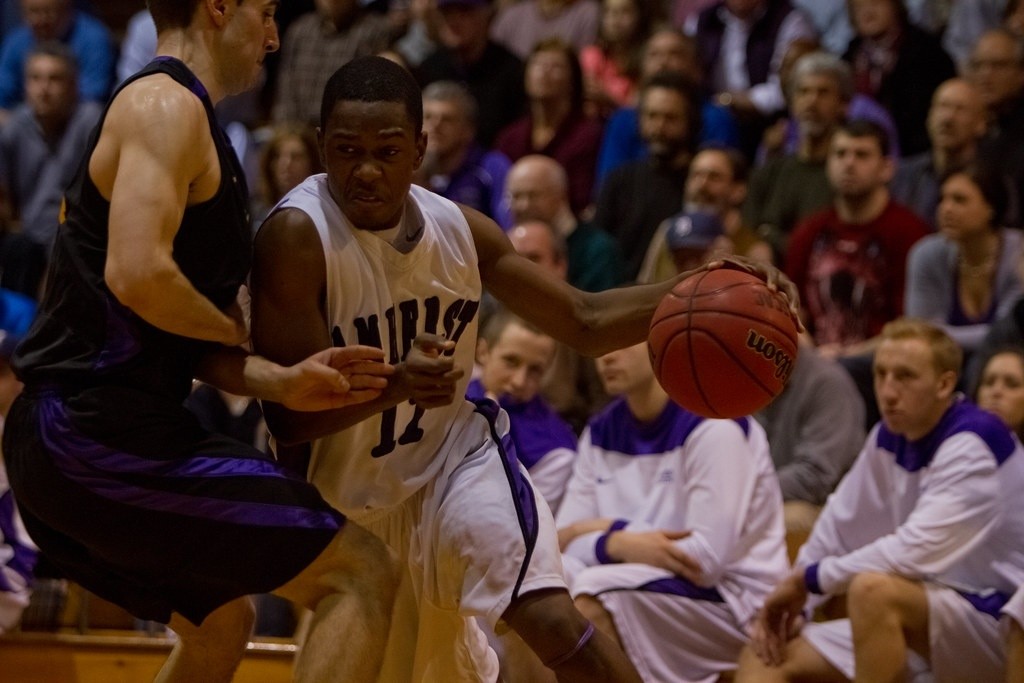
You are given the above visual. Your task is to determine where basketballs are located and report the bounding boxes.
[646,266,801,424]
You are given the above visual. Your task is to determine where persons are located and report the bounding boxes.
[0,0,1022,681]
[1,0,395,682]
[248,55,805,683]
